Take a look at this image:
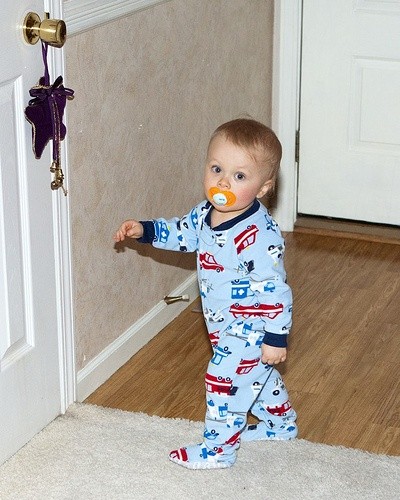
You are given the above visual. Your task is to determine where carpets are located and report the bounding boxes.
[1,402,399,500]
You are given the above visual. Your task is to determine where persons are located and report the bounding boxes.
[112,118,297,471]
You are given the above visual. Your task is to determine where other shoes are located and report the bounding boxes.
[167,444,236,470]
[239,421,298,441]
